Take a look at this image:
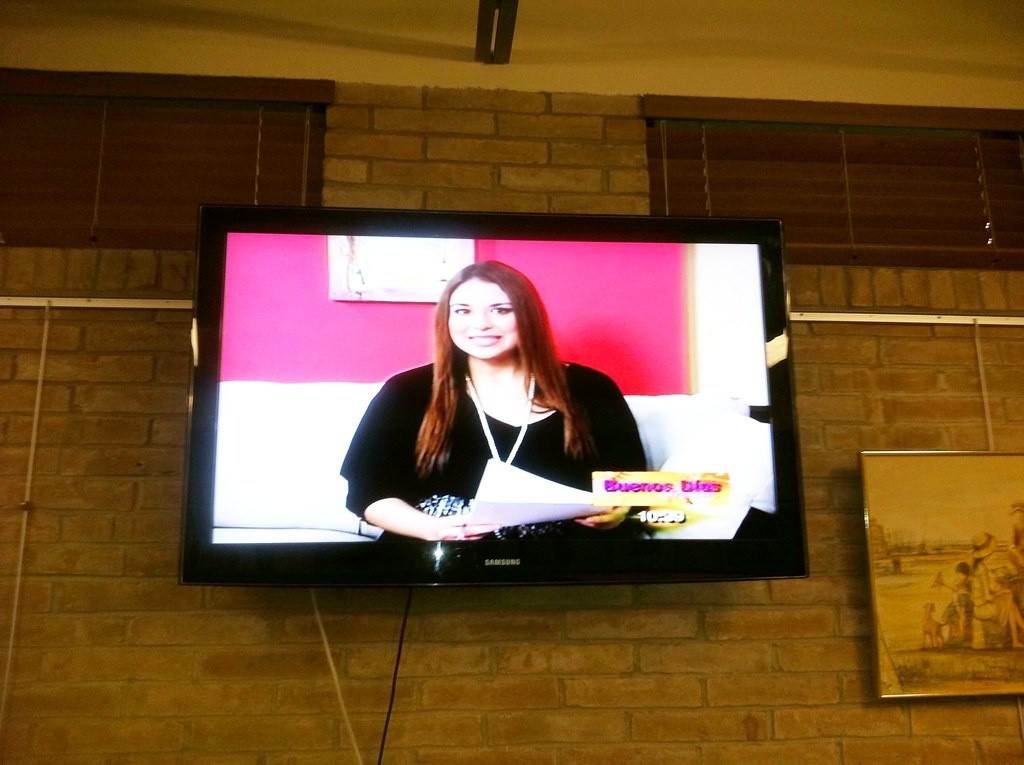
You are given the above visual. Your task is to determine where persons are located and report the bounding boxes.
[342,260,647,542]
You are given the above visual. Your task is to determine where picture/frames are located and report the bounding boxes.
[859,451,1024,701]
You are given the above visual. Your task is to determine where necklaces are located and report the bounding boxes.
[464,373,535,464]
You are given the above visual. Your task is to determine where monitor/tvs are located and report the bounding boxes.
[179,204,810,589]
